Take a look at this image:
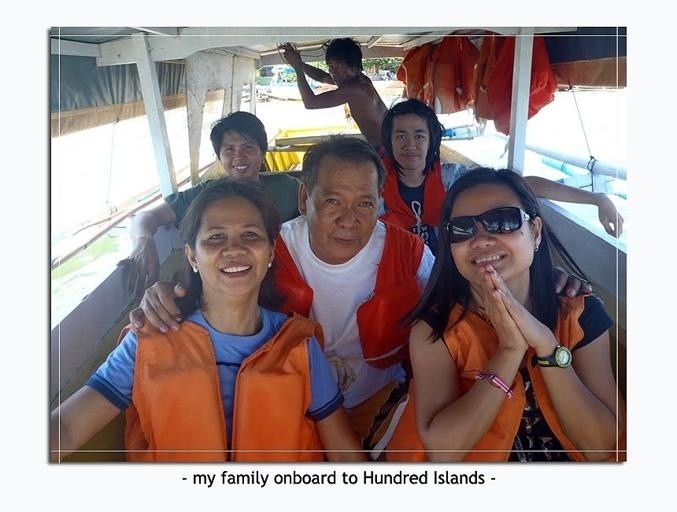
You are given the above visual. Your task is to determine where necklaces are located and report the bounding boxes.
[399,172,427,189]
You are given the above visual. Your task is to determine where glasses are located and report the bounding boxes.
[443,207,530,243]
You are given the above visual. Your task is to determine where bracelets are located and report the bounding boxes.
[133,232,154,242]
[472,368,512,399]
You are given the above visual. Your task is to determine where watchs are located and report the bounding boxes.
[532,343,574,375]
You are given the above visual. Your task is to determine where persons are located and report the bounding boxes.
[129,135,597,462]
[277,37,394,178]
[377,99,624,262]
[382,168,625,460]
[115,109,301,299]
[50,178,370,462]
